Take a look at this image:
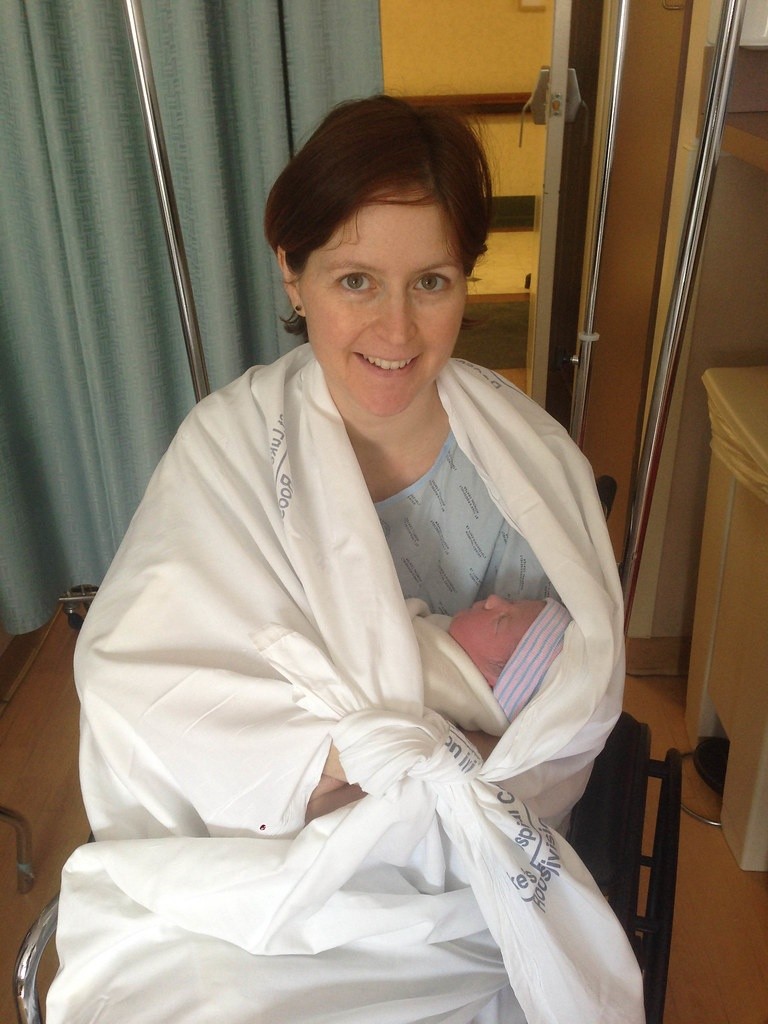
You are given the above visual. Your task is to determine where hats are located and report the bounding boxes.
[493,596,573,723]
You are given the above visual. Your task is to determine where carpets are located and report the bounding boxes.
[450,300,530,370]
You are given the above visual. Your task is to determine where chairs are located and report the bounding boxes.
[17,709,687,1024]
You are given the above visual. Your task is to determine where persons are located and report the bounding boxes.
[405,592,575,737]
[46,92,648,1023]
